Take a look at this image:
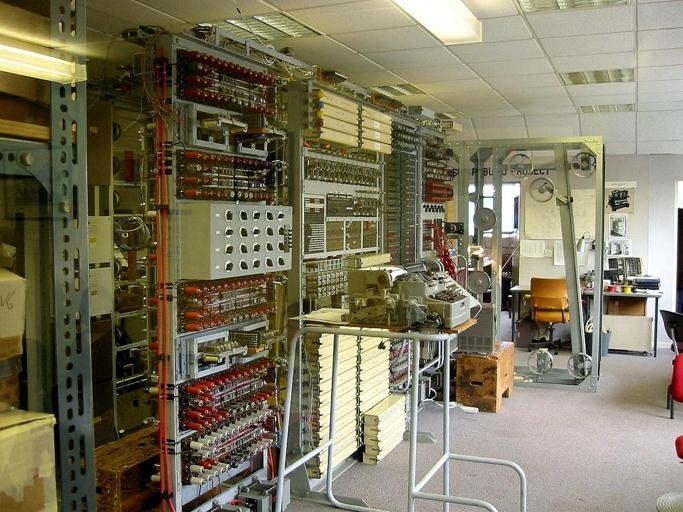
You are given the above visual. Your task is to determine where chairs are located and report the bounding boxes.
[528,277,572,355]
[659,310,683,365]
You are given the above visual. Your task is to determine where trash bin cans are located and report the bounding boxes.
[602,333,609,356]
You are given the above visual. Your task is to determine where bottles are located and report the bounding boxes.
[583,270,595,289]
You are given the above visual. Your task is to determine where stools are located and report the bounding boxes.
[82,426,220,512]
[452,341,514,413]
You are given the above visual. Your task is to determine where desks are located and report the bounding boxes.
[584,287,662,357]
[510,284,587,342]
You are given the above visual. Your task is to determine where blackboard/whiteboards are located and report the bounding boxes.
[601,315,653,355]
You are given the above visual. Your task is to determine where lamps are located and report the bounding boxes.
[393,0,483,46]
